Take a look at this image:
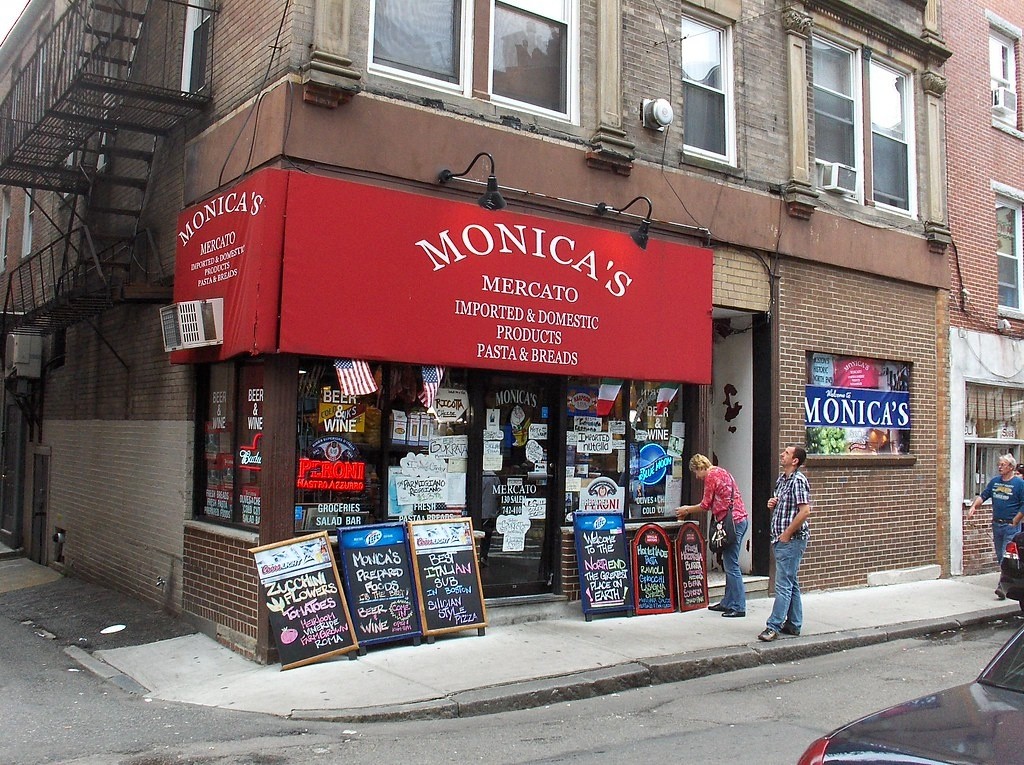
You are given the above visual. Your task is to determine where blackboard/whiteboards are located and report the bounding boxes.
[573,512,634,613]
[629,523,674,614]
[249,530,360,670]
[675,522,708,612]
[408,517,488,635]
[337,522,421,645]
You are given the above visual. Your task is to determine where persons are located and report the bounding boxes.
[966,453,1024,599]
[479,468,502,566]
[758,444,811,642]
[674,454,749,617]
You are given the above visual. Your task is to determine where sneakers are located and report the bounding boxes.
[758,628,777,642]
[780,623,800,635]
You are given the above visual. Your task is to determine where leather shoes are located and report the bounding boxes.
[722,609,746,617]
[708,603,730,612]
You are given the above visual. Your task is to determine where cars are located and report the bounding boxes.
[1000,521,1024,611]
[799,619,1024,765]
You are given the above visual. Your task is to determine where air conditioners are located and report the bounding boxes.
[991,87,1018,117]
[4,334,44,382]
[159,297,224,352]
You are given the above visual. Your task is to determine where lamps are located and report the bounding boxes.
[439,151,508,211]
[598,196,655,252]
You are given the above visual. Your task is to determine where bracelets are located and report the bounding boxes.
[685,506,689,514]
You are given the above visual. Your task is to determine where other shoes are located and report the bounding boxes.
[995,586,1005,599]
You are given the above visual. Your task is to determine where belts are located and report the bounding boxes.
[994,518,1013,524]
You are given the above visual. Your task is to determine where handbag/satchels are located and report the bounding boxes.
[709,505,736,553]
